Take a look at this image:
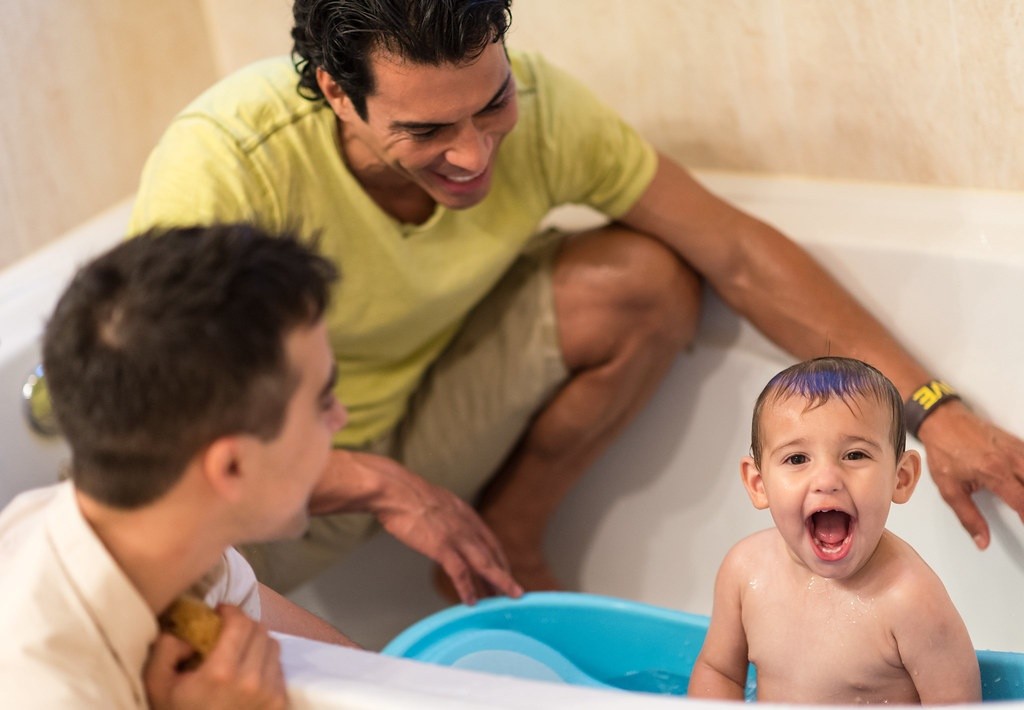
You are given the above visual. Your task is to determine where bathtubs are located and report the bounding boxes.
[0,139,1021,710]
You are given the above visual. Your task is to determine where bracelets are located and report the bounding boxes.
[897,377,963,441]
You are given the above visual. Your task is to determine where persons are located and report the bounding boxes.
[687,356,983,709]
[24,1,1024,602]
[0,221,366,710]
